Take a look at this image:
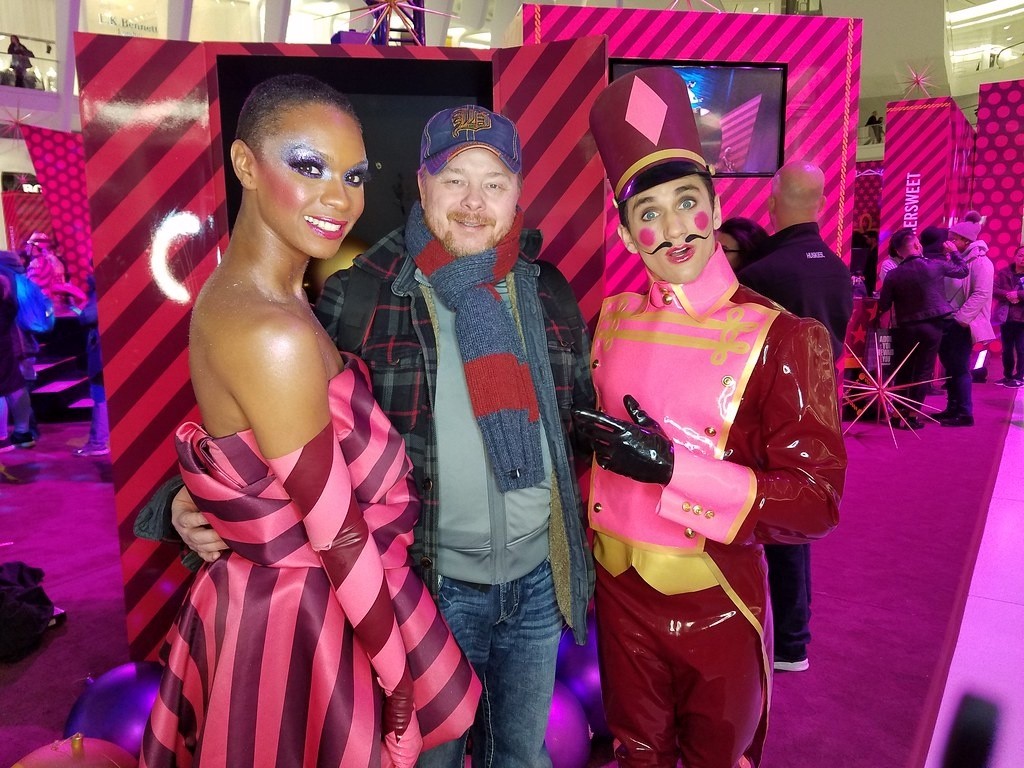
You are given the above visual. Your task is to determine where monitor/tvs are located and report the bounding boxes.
[609,57,788,178]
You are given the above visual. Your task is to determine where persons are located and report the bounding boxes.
[857,231,878,297]
[878,211,996,429]
[686,81,703,103]
[993,247,1024,388]
[734,161,854,671]
[8,34,35,88]
[0,231,74,451]
[717,217,770,271]
[137,102,596,768]
[136,72,485,768]
[71,257,110,456]
[570,156,844,767]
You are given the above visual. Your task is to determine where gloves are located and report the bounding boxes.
[575,397,672,487]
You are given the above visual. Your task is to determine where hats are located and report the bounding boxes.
[590,67,710,203]
[949,212,983,242]
[419,105,523,175]
[919,226,950,247]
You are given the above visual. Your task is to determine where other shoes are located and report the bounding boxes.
[1,426,38,451]
[890,413,925,429]
[995,376,1024,389]
[72,445,109,456]
[933,406,975,426]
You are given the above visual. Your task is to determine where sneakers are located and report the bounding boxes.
[773,640,810,672]
